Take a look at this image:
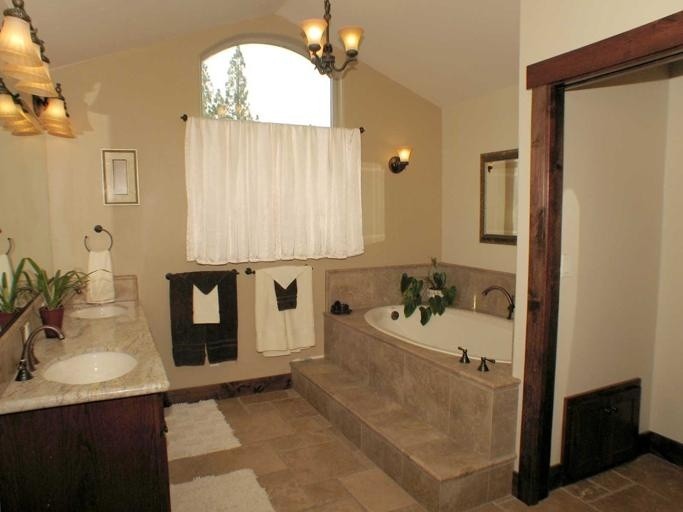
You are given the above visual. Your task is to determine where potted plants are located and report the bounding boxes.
[25,256,111,337]
[399,256,457,325]
[0,254,27,326]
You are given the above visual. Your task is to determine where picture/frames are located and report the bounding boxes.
[100,148,139,207]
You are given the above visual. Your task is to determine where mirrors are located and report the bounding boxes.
[478,149,518,246]
[0,82,56,337]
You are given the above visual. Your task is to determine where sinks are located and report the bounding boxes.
[42,347,139,385]
[70,303,129,319]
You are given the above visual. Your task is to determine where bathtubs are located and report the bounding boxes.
[364,303,514,365]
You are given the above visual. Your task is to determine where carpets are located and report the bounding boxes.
[163,397,241,463]
[167,467,275,511]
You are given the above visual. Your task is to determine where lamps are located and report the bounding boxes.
[31,83,74,141]
[300,1,362,77]
[0,0,58,101]
[388,146,411,173]
[0,79,41,137]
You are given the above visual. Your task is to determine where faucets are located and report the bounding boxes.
[58,284,83,303]
[20,324,65,371]
[10,287,38,312]
[481,285,514,306]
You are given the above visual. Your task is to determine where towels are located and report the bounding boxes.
[85,251,114,304]
